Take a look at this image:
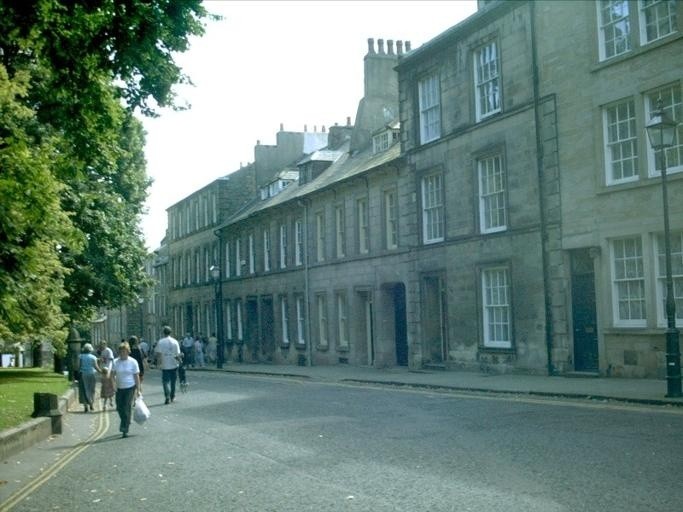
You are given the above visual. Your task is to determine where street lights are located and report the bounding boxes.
[137,297,144,338]
[209,265,222,368]
[645,90,682,399]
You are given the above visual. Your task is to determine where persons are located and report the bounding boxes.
[76,325,219,439]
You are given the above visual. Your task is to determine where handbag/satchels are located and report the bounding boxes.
[73,370,83,380]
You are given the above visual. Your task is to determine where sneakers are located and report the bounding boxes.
[103,403,112,412]
[165,395,175,403]
[123,432,127,437]
[85,406,94,411]
